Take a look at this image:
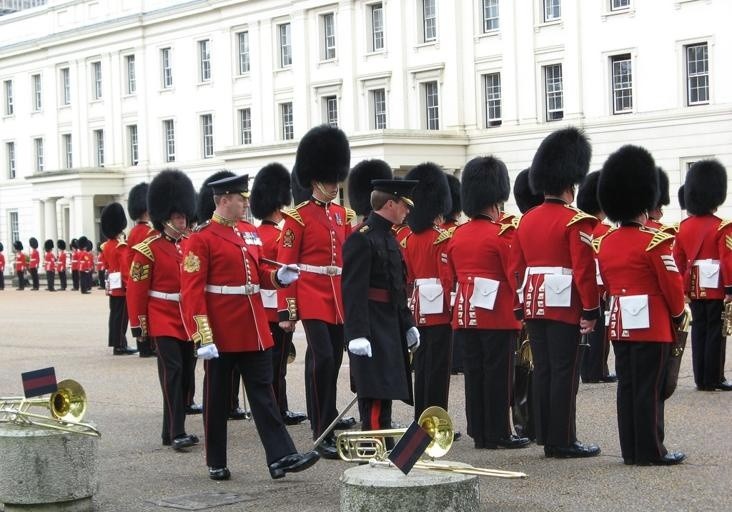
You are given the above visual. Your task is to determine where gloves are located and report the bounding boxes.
[347,336,374,360]
[405,325,423,351]
[191,343,222,363]
[277,262,300,287]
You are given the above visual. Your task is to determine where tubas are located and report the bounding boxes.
[0,379,102,438]
[338,405,528,480]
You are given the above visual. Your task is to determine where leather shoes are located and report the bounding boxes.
[0,284,107,297]
[184,403,205,415]
[624,450,690,466]
[162,434,199,449]
[279,409,308,425]
[268,451,322,479]
[695,376,732,392]
[470,431,531,451]
[227,406,252,422]
[314,435,354,460]
[139,347,159,357]
[451,429,462,443]
[113,343,137,355]
[209,462,232,481]
[580,370,621,384]
[543,439,601,458]
[334,414,357,428]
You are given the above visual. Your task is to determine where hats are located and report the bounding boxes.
[532,127,671,224]
[350,156,545,233]
[101,202,128,241]
[197,123,350,222]
[127,183,150,222]
[684,160,727,216]
[147,168,196,234]
[0,236,106,253]
